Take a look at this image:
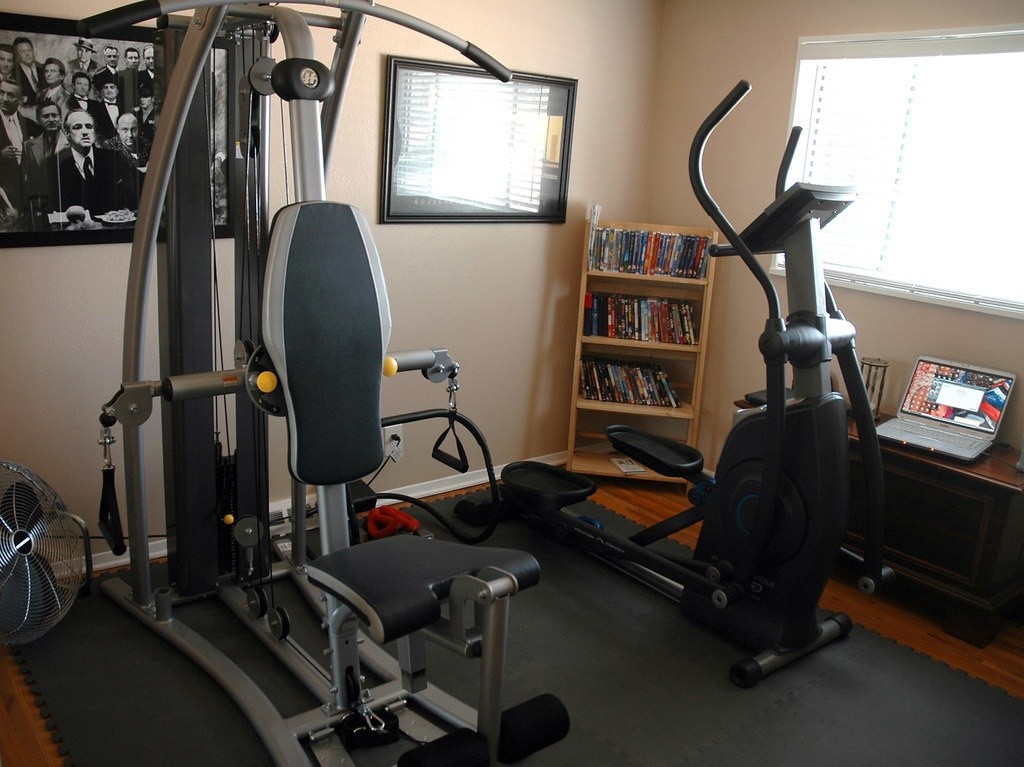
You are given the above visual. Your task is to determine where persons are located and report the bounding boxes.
[0,34,227,229]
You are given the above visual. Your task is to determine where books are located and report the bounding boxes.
[584,292,701,345]
[579,355,682,409]
[590,227,713,280]
[612,458,646,474]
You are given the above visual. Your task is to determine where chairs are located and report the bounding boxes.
[259,197,545,693]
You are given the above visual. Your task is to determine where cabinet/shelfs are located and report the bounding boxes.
[564,201,718,495]
[731,387,1024,649]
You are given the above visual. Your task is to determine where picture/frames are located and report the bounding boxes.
[377,56,579,224]
[0,12,235,248]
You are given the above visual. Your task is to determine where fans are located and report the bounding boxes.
[0,461,82,649]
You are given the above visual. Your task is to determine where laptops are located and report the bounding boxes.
[875,356,1017,465]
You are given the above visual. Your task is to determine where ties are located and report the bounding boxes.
[31,68,38,92]
[83,156,93,184]
[9,115,23,164]
[143,111,148,123]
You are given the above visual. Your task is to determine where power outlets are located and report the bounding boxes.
[384,424,404,457]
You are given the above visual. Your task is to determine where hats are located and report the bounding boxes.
[73,37,97,53]
[137,82,154,97]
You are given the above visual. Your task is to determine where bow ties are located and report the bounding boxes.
[105,102,117,105]
[77,98,88,102]
